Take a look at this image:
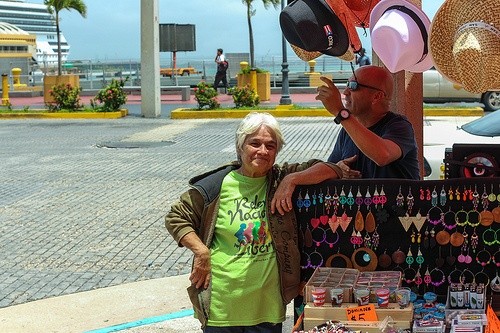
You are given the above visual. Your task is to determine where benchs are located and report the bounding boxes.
[120,85,190,101]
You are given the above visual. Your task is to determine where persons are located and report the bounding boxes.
[315,65,421,279]
[165,111,343,333]
[214,49,229,93]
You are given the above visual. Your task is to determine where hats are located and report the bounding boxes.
[290,44,354,61]
[360,48,366,53]
[325,0,362,53]
[344,0,382,28]
[427,0,500,94]
[280,0,349,57]
[369,0,434,73]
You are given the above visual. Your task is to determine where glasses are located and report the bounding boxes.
[347,81,386,97]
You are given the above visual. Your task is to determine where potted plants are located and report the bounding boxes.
[44,0,86,103]
[238,0,279,100]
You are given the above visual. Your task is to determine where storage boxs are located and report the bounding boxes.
[301,266,487,333]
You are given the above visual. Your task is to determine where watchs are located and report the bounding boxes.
[334,109,352,124]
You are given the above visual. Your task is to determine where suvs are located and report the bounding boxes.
[423,66,500,111]
